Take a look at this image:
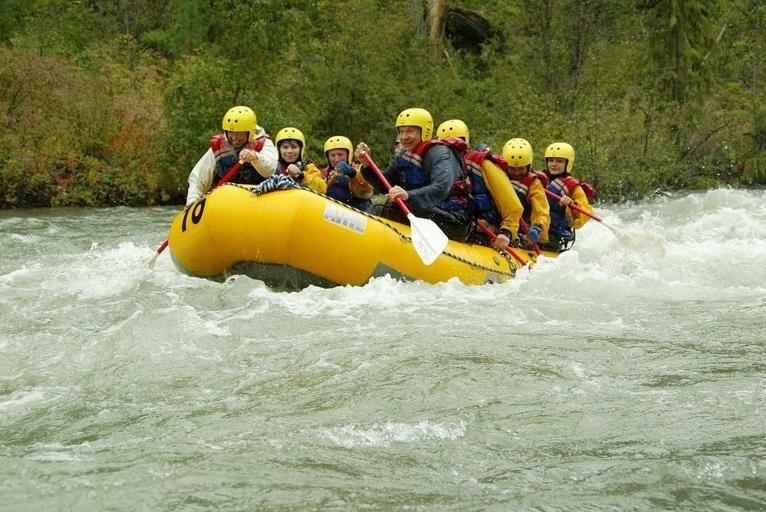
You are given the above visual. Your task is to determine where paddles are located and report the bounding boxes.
[544,189,636,243]
[361,151,449,265]
[140,161,245,271]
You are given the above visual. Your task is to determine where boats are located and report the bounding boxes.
[167,183,562,293]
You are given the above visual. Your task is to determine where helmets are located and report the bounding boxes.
[276,127,305,157]
[503,138,533,172]
[545,142,574,173]
[436,119,469,146]
[396,108,433,142]
[222,106,257,143]
[323,136,354,164]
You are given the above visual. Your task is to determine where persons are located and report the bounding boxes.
[434,118,525,252]
[537,142,596,258]
[184,105,280,207]
[274,126,327,195]
[316,135,374,212]
[354,107,471,244]
[501,137,552,251]
